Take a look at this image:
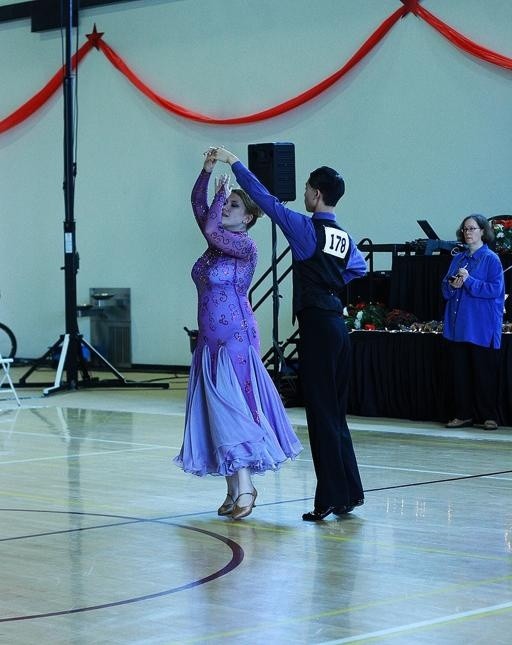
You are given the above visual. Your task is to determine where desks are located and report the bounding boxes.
[346,331,512,427]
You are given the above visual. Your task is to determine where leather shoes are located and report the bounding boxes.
[302,499,364,521]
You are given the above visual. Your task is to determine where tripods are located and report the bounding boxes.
[255,211,303,406]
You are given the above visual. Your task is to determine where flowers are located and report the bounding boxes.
[344,301,386,329]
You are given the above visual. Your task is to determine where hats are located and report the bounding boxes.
[310,166,344,199]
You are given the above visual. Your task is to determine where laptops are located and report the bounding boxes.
[417,220,441,240]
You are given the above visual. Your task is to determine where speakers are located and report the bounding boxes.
[248,140,296,203]
[26,0,80,33]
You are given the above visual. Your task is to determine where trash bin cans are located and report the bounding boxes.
[89,287,132,368]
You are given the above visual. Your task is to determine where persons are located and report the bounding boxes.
[440,213,505,429]
[176,145,304,522]
[204,144,370,525]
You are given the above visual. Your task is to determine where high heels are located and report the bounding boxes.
[445,418,472,427]
[218,488,258,520]
[484,420,497,430]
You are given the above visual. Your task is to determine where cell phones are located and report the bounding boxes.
[447,276,457,283]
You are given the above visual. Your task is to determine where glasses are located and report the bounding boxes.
[461,226,482,233]
